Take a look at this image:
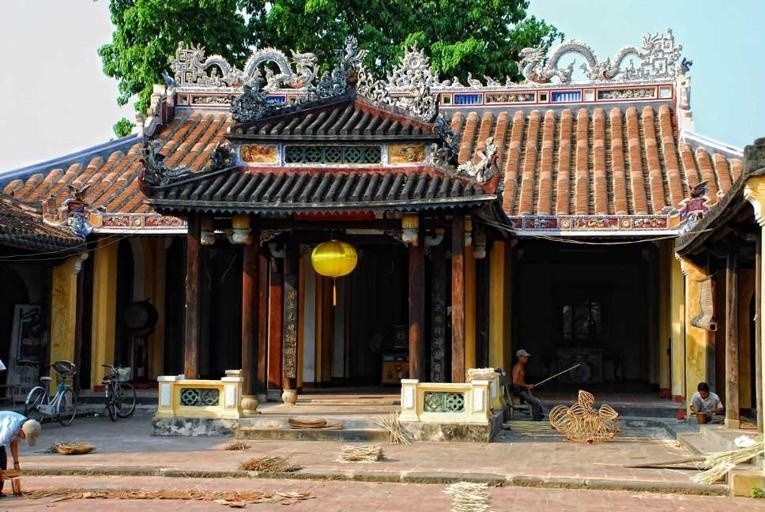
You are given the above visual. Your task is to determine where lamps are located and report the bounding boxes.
[225,228,236,245]
[401,213,419,242]
[201,219,215,245]
[473,231,488,259]
[231,215,250,243]
[464,214,473,247]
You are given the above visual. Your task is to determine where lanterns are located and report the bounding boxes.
[311,229,359,307]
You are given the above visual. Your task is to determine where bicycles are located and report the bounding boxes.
[24,364,81,428]
[101,364,136,422]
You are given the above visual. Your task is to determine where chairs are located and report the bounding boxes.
[505,384,533,417]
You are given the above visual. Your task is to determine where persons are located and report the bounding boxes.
[512,349,544,421]
[690,382,723,423]
[0,409,42,497]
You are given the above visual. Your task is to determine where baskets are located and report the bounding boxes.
[111,368,131,381]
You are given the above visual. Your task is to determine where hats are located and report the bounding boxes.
[517,350,530,356]
[22,419,41,447]
